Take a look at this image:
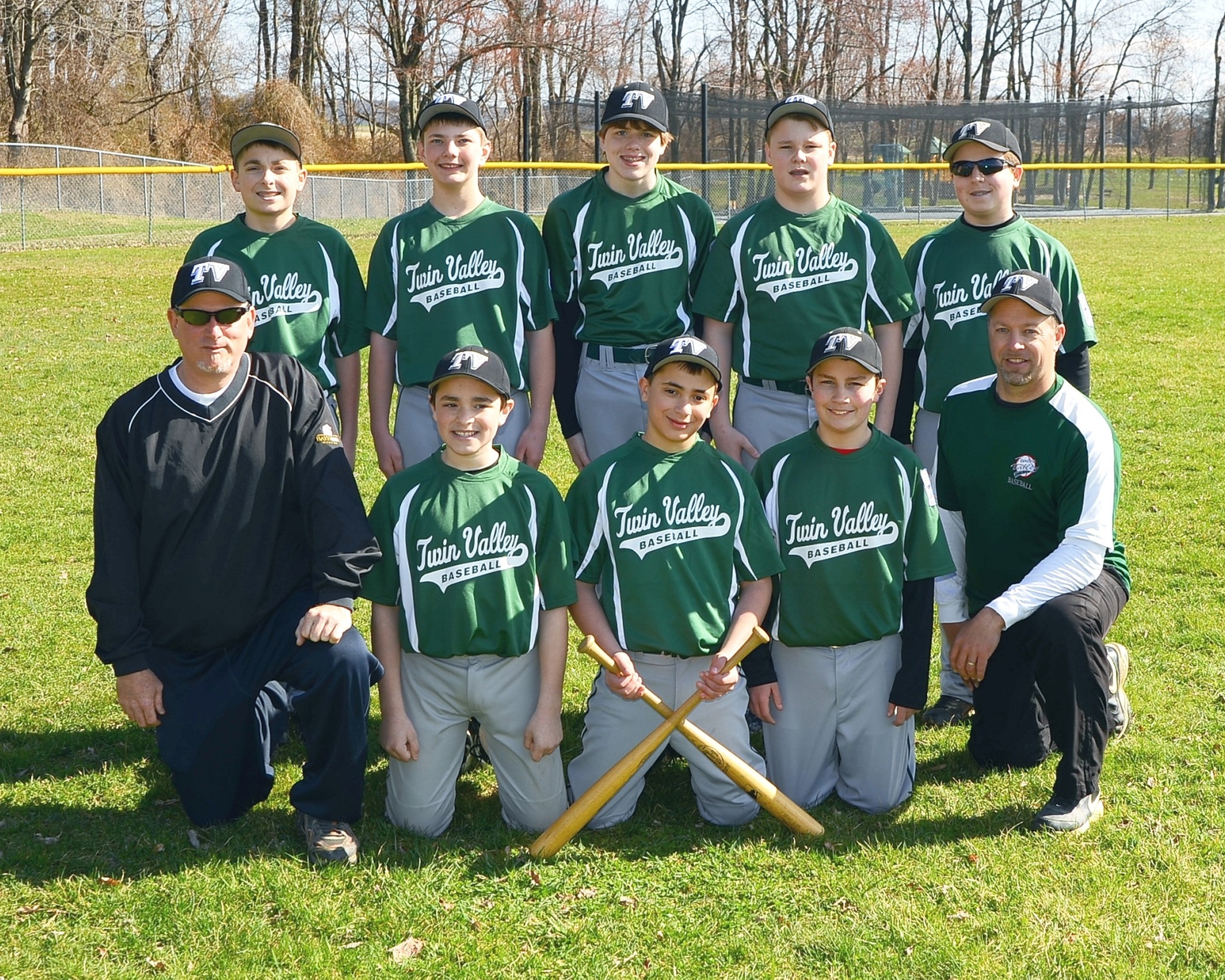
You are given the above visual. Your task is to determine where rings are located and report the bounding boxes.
[967,660,975,666]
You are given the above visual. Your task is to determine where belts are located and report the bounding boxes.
[581,342,660,365]
[741,375,813,400]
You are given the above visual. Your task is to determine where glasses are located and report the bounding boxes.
[949,157,1016,177]
[173,303,252,326]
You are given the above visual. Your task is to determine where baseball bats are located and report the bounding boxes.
[578,634,824,840]
[530,623,772,861]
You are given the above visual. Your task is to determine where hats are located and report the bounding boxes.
[170,255,253,309]
[765,94,835,142]
[941,117,1022,166]
[428,344,512,401]
[804,326,883,382]
[230,120,303,171]
[416,92,488,139]
[979,268,1064,325]
[642,335,723,394]
[600,81,670,132]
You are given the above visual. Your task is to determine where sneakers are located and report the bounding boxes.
[920,694,974,728]
[1104,641,1132,741]
[474,719,493,767]
[1030,784,1105,837]
[294,807,361,867]
[460,730,473,773]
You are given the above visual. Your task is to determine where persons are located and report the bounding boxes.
[360,345,578,841]
[892,117,1101,454]
[690,94,919,472]
[184,122,370,478]
[86,255,385,866]
[366,91,560,484]
[741,325,956,818]
[932,267,1134,835]
[542,82,715,469]
[564,337,787,830]
